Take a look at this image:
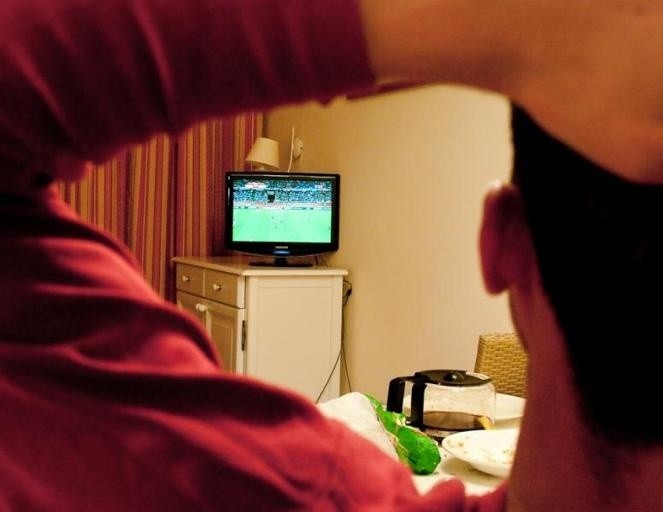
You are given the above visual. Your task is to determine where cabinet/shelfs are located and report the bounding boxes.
[170,256,349,405]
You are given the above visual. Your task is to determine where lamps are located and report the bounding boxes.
[244,137,279,170]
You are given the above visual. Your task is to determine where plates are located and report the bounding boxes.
[441,428,519,479]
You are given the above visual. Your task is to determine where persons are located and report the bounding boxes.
[0,1,662,511]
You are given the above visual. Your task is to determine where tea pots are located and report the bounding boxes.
[386,367,497,444]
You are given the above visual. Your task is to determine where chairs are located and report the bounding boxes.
[473,334,528,397]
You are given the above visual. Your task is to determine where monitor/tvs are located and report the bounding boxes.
[222,170,341,269]
